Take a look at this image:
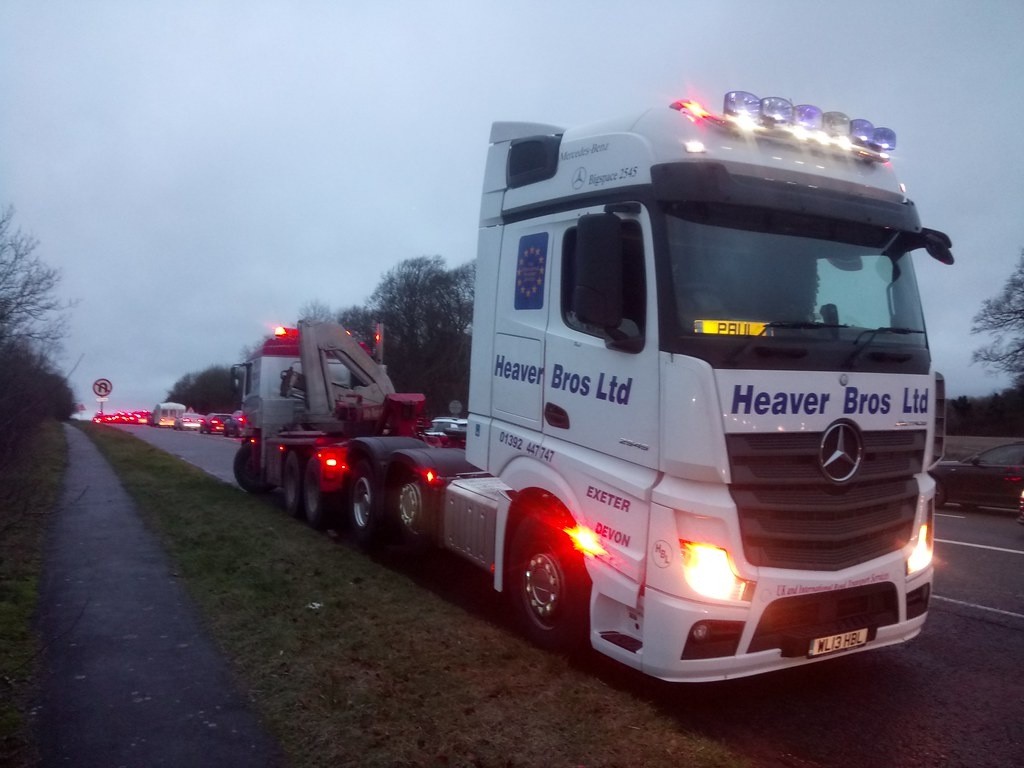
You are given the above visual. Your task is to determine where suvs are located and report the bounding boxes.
[418,416,469,438]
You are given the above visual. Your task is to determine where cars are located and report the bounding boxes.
[223,409,256,439]
[173,413,205,432]
[927,438,1024,512]
[92,409,152,428]
[199,413,233,435]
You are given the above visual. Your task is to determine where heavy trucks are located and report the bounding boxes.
[228,88,958,689]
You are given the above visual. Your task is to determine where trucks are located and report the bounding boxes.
[152,401,187,428]
